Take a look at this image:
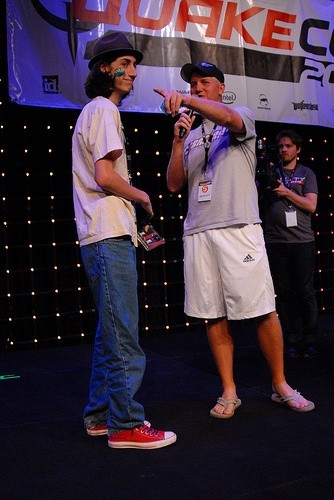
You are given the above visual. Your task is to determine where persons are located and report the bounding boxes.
[152,61,316,419]
[71,29,178,450]
[253,134,320,360]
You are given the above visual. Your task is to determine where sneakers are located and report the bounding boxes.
[87,417,151,436]
[108,427,177,450]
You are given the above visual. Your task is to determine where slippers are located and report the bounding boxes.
[210,394,241,418]
[271,389,315,412]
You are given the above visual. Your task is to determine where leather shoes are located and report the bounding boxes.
[304,344,318,357]
[286,341,300,356]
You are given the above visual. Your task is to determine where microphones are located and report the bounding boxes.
[179,94,199,139]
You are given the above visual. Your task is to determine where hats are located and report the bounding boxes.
[88,31,143,70]
[180,61,224,83]
